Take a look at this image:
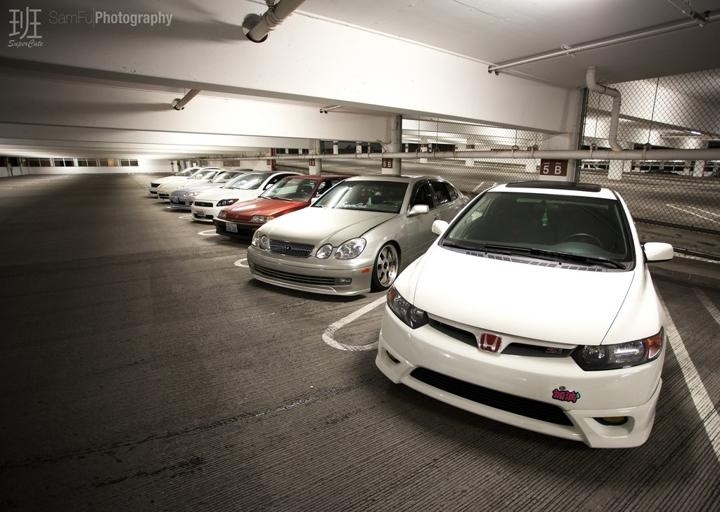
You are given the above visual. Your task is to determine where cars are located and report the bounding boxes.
[149,160,239,213]
[245,170,469,298]
[190,168,296,226]
[215,174,351,246]
[375,179,674,451]
